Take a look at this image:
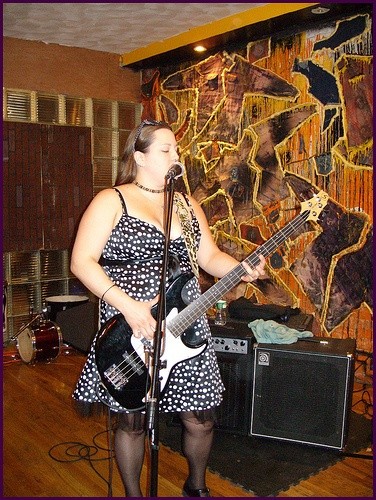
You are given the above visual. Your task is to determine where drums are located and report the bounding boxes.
[17,319,63,365]
[44,294,91,323]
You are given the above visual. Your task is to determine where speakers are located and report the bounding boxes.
[248,337,357,452]
[167,322,256,435]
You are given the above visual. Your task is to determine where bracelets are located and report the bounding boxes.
[101,284,117,300]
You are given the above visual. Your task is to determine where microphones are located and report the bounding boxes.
[165,162,184,179]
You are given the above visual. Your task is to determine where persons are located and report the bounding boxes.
[70,120,266,497]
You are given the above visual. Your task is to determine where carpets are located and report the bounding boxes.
[153,409,373,497]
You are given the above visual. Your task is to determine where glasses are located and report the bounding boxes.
[131,118,169,152]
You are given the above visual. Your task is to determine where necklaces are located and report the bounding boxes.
[133,180,169,193]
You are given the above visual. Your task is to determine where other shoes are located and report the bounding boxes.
[182,481,211,497]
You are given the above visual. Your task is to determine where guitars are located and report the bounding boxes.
[93,187,331,412]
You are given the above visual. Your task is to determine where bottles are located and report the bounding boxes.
[214,296,227,326]
